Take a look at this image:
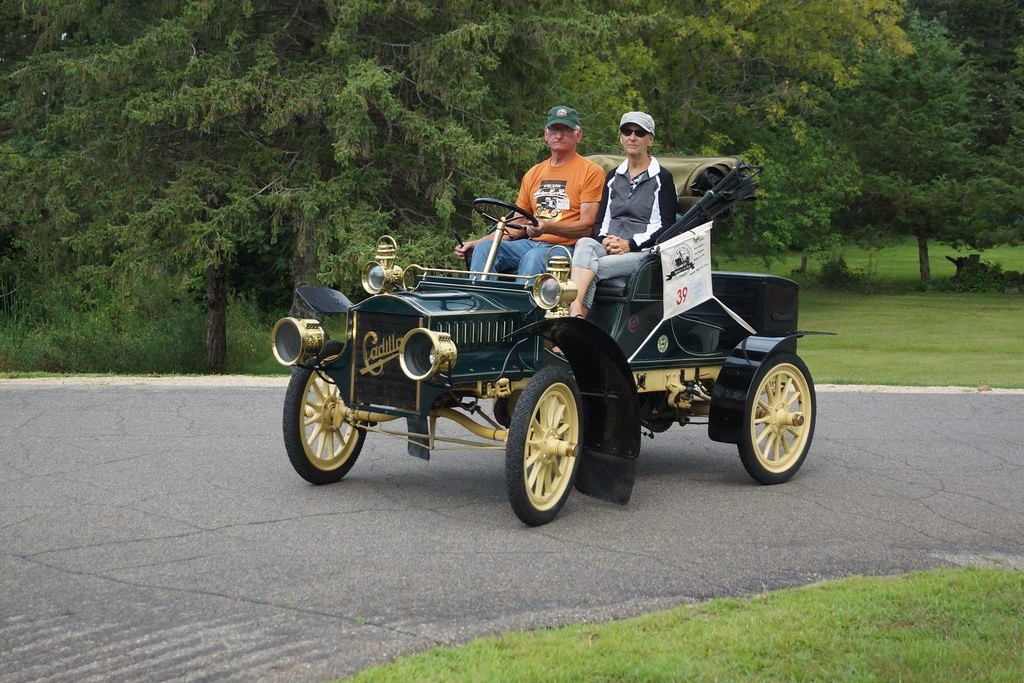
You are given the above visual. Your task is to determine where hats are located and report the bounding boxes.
[618,112,656,136]
[546,106,580,129]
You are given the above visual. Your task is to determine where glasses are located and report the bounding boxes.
[548,126,576,135]
[620,128,652,137]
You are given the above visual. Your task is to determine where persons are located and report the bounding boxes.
[455,106,607,284]
[570,111,677,319]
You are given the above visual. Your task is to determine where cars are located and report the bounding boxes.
[270,156,842,528]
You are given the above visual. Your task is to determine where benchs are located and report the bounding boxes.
[499,196,703,302]
